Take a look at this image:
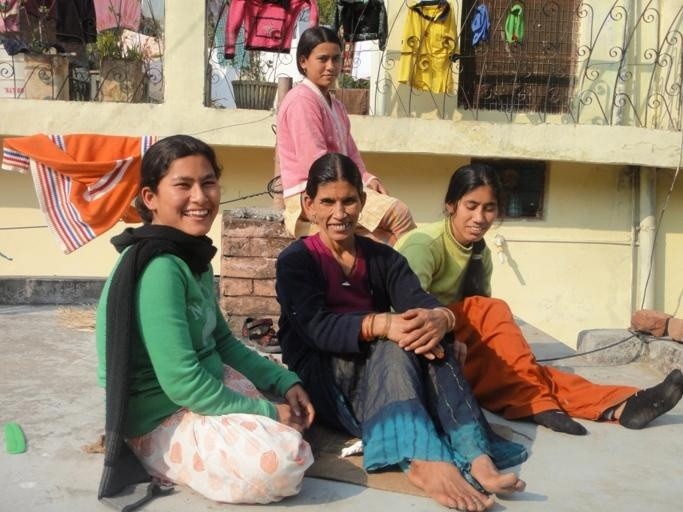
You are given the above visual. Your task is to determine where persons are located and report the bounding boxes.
[272,149,527,512]
[92,134,319,504]
[394,162,683,438]
[273,27,417,256]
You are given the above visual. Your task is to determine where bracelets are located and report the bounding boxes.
[440,304,456,335]
[364,312,376,344]
[381,311,392,338]
[434,307,451,331]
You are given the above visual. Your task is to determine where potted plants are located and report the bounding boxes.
[334,73,370,115]
[231,55,278,110]
[88,32,149,103]
[22,6,72,101]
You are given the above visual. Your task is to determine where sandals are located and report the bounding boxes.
[242,317,282,352]
